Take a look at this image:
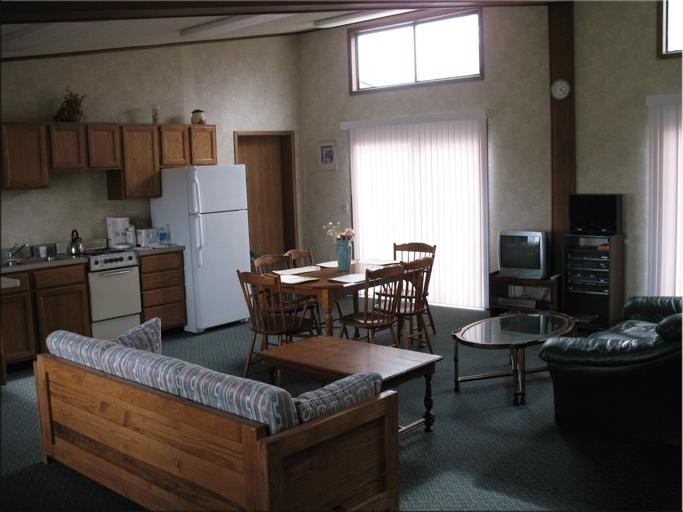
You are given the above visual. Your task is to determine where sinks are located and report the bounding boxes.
[28,256,68,264]
[1,259,27,268]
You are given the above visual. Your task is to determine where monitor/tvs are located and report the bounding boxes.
[496,229,549,280]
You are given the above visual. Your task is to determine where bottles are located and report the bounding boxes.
[125,222,168,250]
[189,109,206,124]
[151,103,160,123]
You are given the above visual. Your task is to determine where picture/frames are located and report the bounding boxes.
[317,142,336,170]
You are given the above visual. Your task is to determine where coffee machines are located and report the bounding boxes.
[105,216,130,250]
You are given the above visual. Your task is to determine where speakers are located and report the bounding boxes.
[567,194,623,234]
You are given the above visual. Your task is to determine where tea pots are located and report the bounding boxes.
[67,228,85,256]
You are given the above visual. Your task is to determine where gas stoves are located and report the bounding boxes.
[81,247,140,272]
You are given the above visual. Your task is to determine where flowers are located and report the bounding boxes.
[322,221,355,244]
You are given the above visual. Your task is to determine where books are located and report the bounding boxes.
[570,244,610,325]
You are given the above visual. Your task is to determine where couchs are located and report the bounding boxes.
[34,316,401,512]
[537,296,682,434]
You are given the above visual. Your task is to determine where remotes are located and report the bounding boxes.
[549,274,561,280]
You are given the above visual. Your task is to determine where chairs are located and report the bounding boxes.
[234,243,438,380]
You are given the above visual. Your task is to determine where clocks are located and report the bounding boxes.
[549,78,572,102]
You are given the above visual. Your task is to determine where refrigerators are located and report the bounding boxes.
[148,163,254,335]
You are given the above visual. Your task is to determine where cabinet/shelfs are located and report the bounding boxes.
[0,260,90,381]
[105,125,160,198]
[49,124,122,172]
[0,118,48,191]
[140,249,187,334]
[488,272,558,325]
[561,235,624,329]
[159,127,216,167]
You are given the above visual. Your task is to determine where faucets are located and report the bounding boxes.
[9,241,30,260]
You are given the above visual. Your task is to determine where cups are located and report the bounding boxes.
[37,245,47,258]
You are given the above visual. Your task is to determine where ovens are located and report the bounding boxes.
[86,265,141,341]
